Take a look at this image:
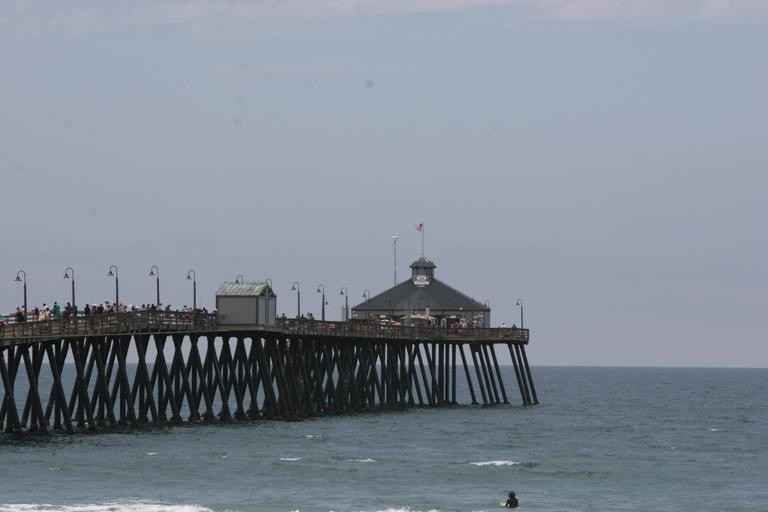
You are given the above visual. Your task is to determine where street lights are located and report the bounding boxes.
[108,266,118,312]
[64,267,75,306]
[292,281,300,317]
[186,270,196,308]
[363,289,370,320]
[150,266,159,306]
[517,299,523,328]
[457,300,488,329]
[16,270,27,323]
[340,288,348,323]
[317,284,324,320]
[384,291,430,326]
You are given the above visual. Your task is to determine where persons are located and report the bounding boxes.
[278,312,315,330]
[512,324,517,328]
[505,488,518,507]
[501,323,506,328]
[0,302,217,335]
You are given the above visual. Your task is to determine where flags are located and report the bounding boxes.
[416,223,422,232]
